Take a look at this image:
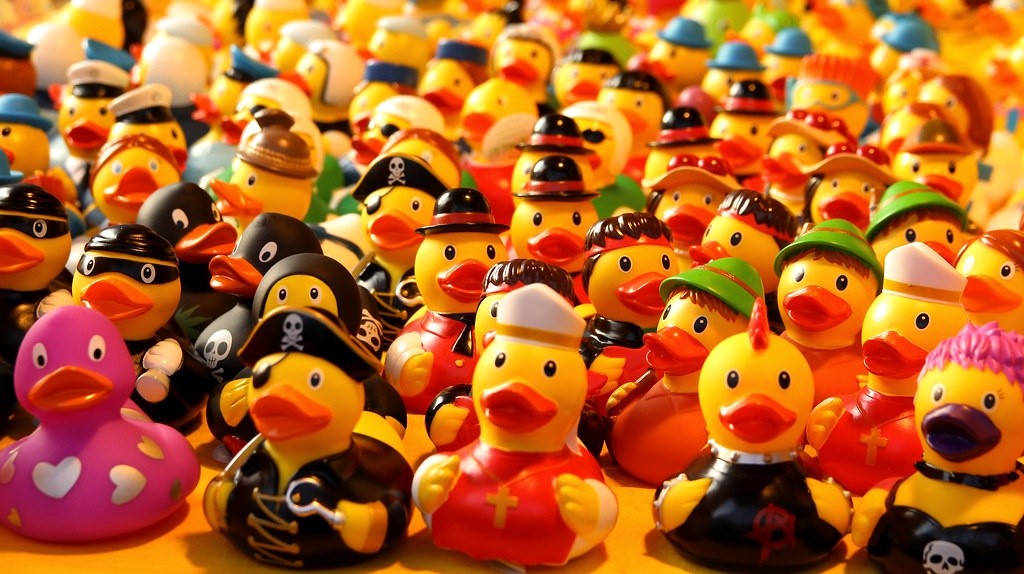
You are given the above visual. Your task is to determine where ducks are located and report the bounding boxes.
[0,0,1024,574]
